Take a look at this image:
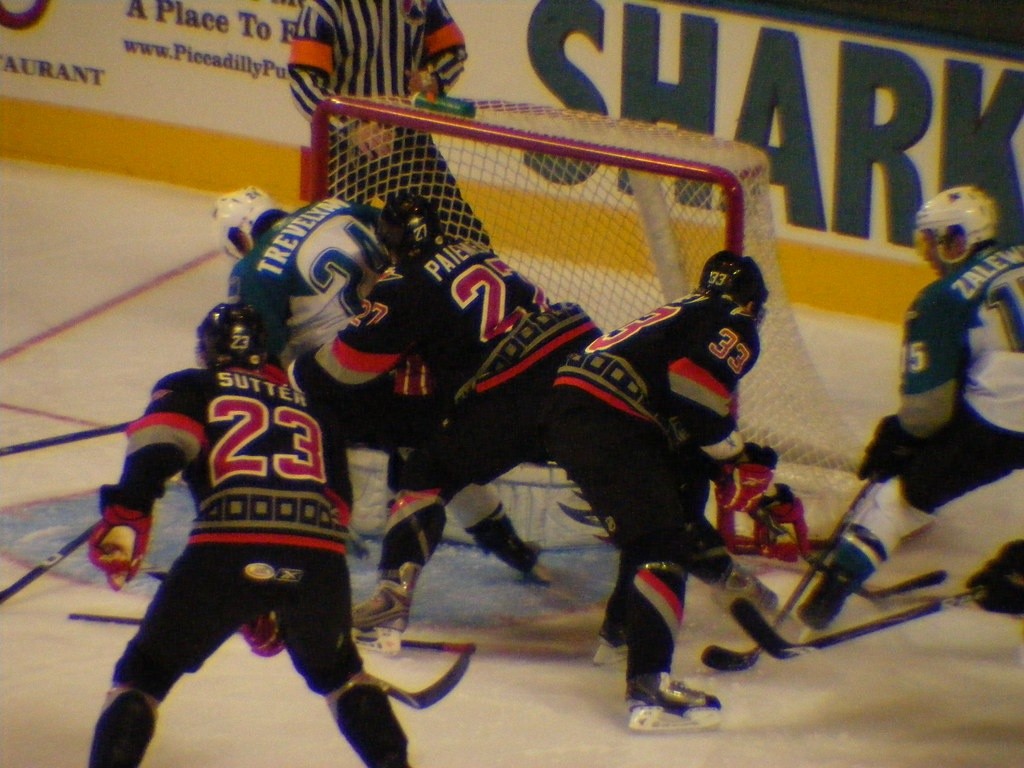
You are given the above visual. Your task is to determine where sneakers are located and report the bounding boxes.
[511,539,557,587]
[592,598,630,665]
[793,570,853,646]
[625,665,721,732]
[967,542,1024,617]
[349,561,423,657]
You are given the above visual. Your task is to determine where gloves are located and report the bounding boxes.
[754,483,812,562]
[90,483,153,592]
[714,461,773,515]
[242,607,286,657]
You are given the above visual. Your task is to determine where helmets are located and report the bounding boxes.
[700,250,768,305]
[916,184,996,264]
[197,301,270,370]
[379,192,443,252]
[212,184,275,260]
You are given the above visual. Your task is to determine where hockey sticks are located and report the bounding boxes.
[700,477,873,674]
[110,548,472,713]
[1,419,133,456]
[1,515,107,604]
[706,462,950,603]
[726,582,988,663]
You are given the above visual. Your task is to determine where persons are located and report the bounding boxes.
[86,305,408,767]
[288,0,491,250]
[551,251,808,734]
[210,186,777,659]
[799,186,1024,640]
